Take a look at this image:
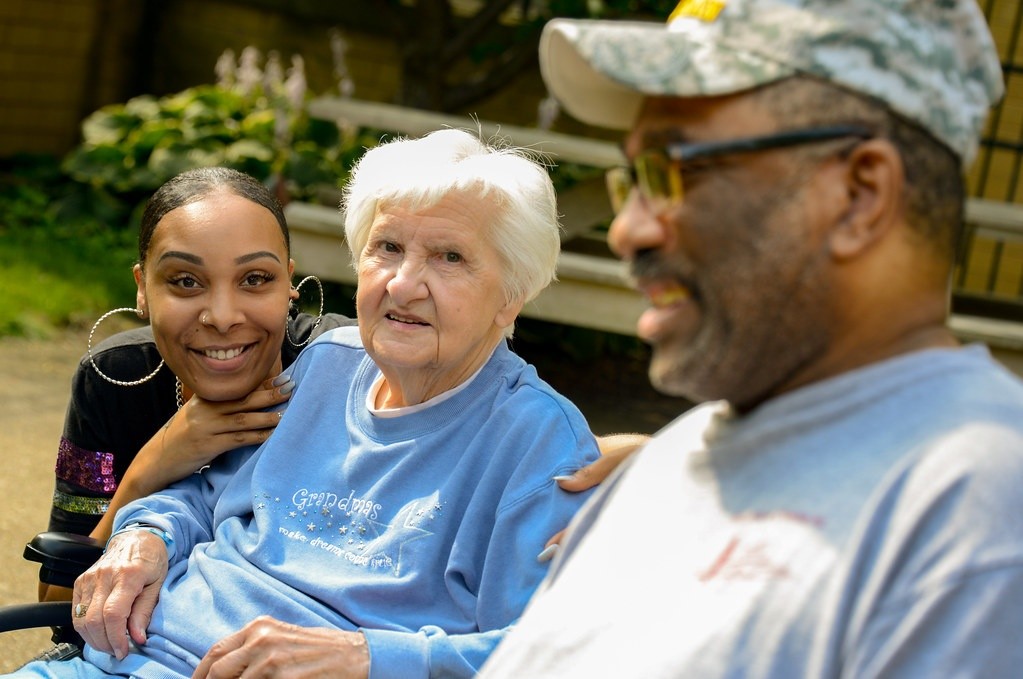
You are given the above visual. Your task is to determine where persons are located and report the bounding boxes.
[0,128,602,678]
[38,165,651,647]
[461,0,1023,679]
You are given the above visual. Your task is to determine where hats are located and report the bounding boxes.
[539,0,1004,167]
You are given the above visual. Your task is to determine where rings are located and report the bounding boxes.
[73,603,87,617]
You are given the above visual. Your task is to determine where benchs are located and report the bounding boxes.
[278,101,1022,388]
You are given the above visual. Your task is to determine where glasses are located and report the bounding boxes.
[603,123,876,218]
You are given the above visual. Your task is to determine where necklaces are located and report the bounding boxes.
[175,376,184,410]
